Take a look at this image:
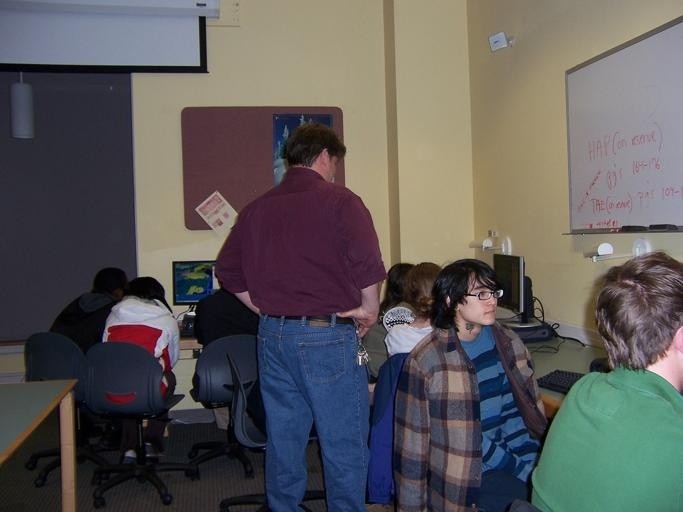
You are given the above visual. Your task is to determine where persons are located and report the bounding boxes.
[50,267,177,458]
[192,271,267,436]
[531,252,681,511]
[362,262,443,380]
[214,124,388,510]
[392,258,550,510]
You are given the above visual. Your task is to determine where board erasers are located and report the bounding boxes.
[622,224,678,231]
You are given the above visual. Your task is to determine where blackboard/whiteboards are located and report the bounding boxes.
[566,15,683,235]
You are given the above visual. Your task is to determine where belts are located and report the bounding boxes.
[262,310,355,325]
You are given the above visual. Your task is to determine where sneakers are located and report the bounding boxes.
[84,439,170,465]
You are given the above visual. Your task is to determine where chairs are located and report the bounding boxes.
[82,339,199,510]
[183,331,266,483]
[219,350,329,512]
[21,331,107,488]
[507,497,544,512]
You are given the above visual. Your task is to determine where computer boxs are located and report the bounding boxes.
[496,317,553,342]
[181,312,195,331]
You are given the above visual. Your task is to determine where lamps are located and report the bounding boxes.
[467,237,515,258]
[580,236,654,266]
[8,71,37,143]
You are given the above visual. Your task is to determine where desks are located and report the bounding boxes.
[524,337,609,420]
[179,336,205,351]
[1,377,81,512]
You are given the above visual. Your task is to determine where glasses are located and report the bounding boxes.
[463,288,504,300]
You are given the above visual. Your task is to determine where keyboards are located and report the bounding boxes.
[536,369,586,395]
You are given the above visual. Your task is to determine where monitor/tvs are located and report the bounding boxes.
[492,252,543,328]
[172,260,221,306]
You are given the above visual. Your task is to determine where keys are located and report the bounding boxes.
[356,345,368,366]
